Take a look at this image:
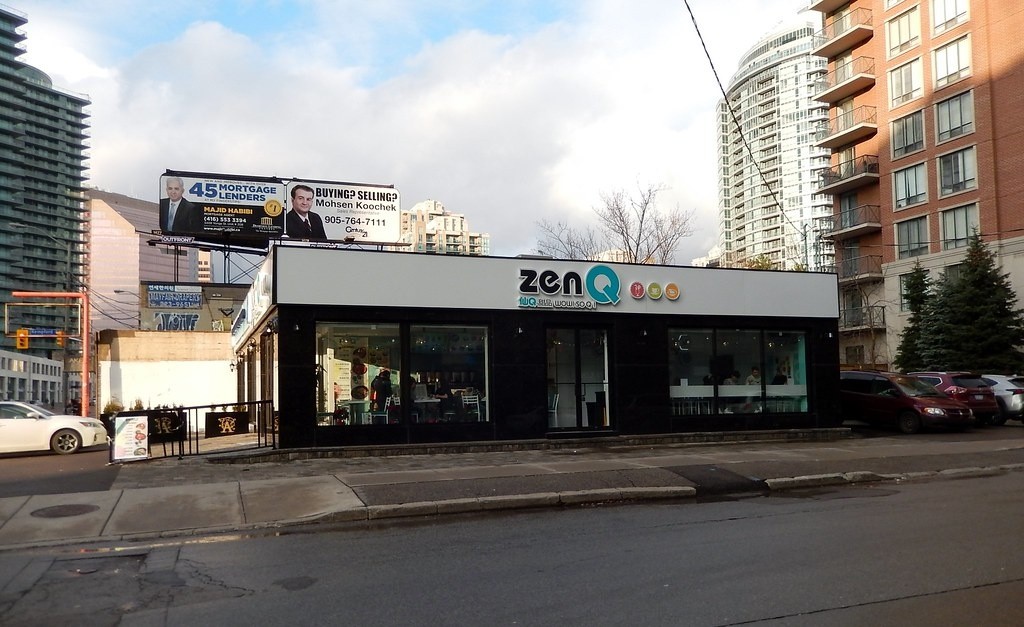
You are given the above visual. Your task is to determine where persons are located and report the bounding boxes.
[432,378,456,414]
[160,178,196,232]
[719,371,743,414]
[373,369,393,408]
[745,367,763,413]
[285,185,328,240]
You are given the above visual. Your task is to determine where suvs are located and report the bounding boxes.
[980,373,1024,427]
[906,372,999,428]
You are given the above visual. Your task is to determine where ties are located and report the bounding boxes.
[168,203,176,231]
[304,219,310,230]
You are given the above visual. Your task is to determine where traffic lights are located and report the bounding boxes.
[56,331,64,347]
[17,329,28,349]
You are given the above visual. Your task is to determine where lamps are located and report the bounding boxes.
[518,327,522,334]
[230,349,245,372]
[266,321,278,337]
[643,329,647,336]
[247,337,259,356]
[829,332,832,338]
[294,319,300,331]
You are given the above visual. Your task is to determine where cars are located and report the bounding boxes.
[24,400,45,407]
[89,399,96,406]
[840,368,976,433]
[726,390,802,412]
[64,399,82,414]
[0,400,108,458]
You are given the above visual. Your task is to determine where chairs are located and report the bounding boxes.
[444,395,484,422]
[548,394,559,427]
[671,397,710,416]
[372,397,391,424]
[316,412,334,425]
[394,397,418,423]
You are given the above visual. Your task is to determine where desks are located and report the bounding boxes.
[670,385,807,397]
[414,399,440,423]
[348,401,372,425]
